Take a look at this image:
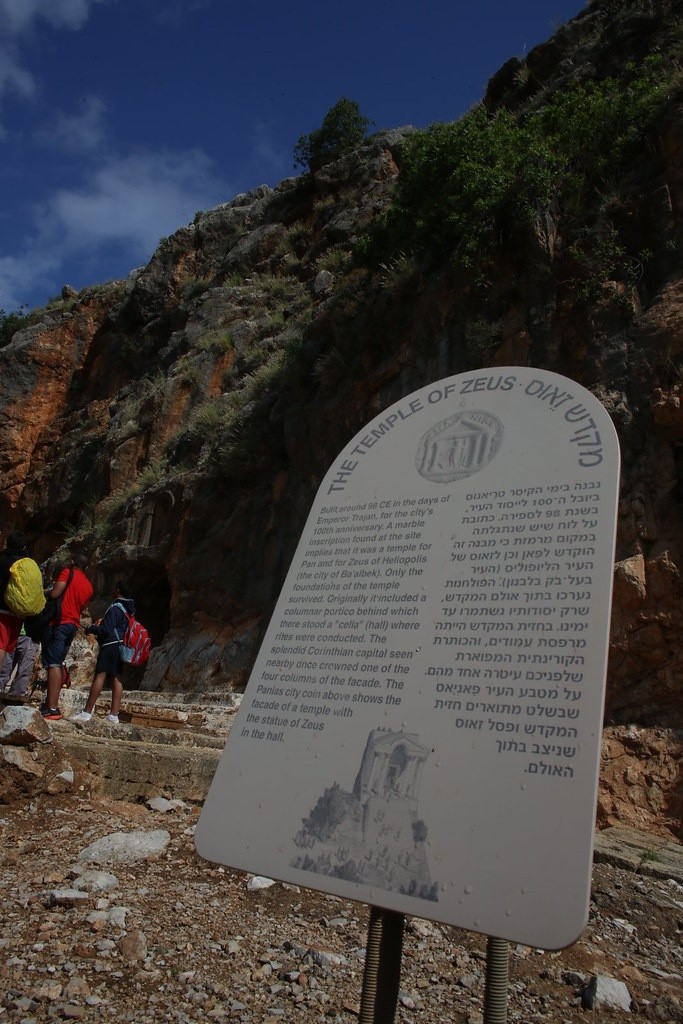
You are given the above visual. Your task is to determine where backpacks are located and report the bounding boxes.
[3,551,47,618]
[104,602,151,667]
[23,566,74,646]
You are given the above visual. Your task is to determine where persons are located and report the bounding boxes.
[0,526,40,706]
[42,555,94,721]
[68,578,137,725]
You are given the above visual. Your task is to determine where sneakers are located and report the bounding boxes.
[38,703,63,720]
[102,716,120,725]
[68,710,91,721]
